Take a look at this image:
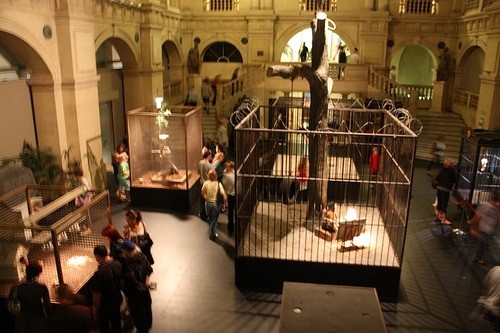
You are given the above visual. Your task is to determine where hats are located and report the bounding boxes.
[122,241,134,250]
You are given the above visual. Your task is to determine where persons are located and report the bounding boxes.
[199,152,213,218]
[434,158,455,224]
[332,43,360,78]
[18,263,98,333]
[213,152,226,212]
[299,41,309,64]
[223,163,242,232]
[201,66,239,114]
[203,145,225,163]
[369,147,380,188]
[74,170,92,235]
[200,170,228,241]
[468,266,500,333]
[472,195,500,265]
[93,209,154,333]
[390,65,398,98]
[111,144,129,189]
[328,111,340,128]
[187,83,205,110]
[117,155,131,200]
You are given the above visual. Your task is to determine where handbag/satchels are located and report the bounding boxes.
[216,182,224,206]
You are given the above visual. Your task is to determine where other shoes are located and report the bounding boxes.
[213,233,218,241]
[442,220,452,225]
